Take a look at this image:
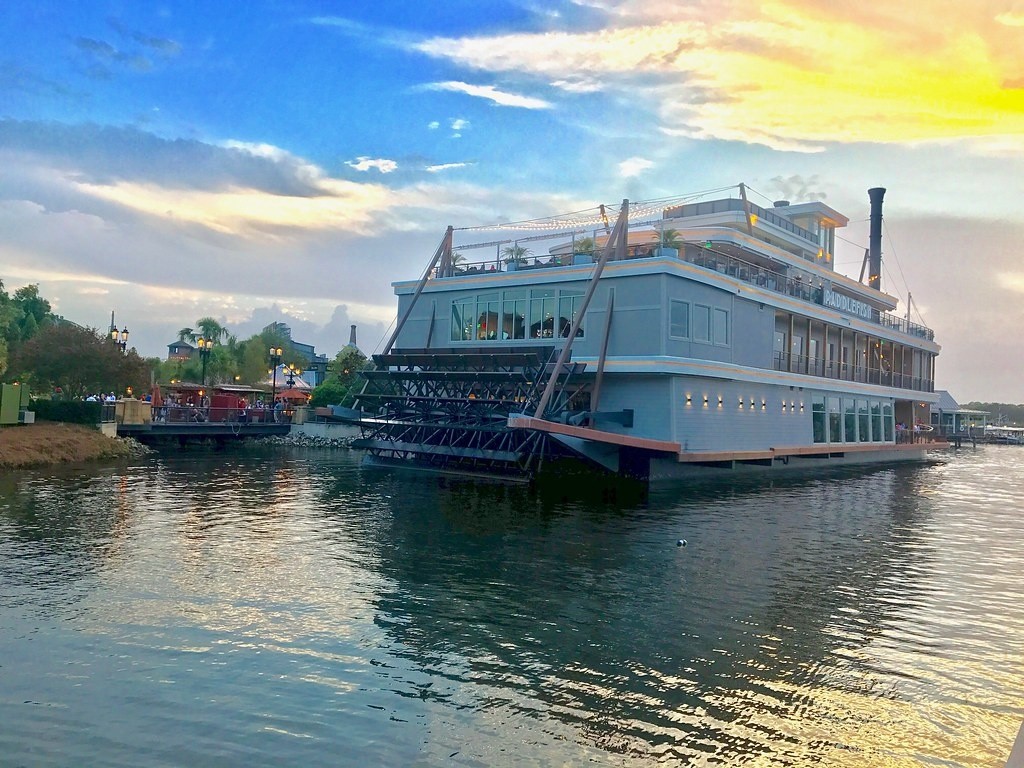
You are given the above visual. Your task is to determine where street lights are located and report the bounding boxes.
[110,325,129,393]
[270,345,283,409]
[197,336,214,408]
[282,362,302,390]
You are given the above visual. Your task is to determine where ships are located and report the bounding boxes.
[314,181,951,487]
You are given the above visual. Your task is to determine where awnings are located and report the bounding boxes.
[151,383,208,407]
[210,384,265,408]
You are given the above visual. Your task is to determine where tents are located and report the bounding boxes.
[276,387,308,406]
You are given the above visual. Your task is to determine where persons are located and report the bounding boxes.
[51,383,292,425]
[895,420,922,445]
